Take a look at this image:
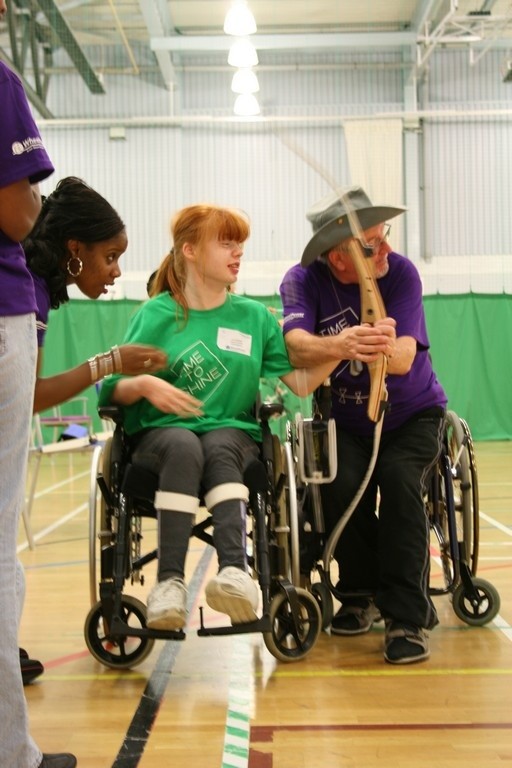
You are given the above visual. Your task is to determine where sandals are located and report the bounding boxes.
[384,617,430,664]
[331,598,381,633]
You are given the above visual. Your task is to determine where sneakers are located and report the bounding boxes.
[146,575,188,630]
[205,563,265,625]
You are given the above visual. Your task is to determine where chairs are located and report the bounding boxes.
[34,397,92,442]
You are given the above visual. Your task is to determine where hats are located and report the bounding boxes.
[300,186,407,266]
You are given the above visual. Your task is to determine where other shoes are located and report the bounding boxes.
[38,752,77,768]
[18,647,44,686]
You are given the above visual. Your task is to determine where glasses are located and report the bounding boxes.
[337,222,394,252]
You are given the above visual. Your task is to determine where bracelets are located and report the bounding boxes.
[87,345,123,383]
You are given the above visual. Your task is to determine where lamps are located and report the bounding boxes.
[223,0,261,115]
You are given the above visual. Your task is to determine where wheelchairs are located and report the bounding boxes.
[288,412,502,631]
[82,404,321,671]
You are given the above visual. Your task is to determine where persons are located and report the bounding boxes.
[98,203,370,635]
[19,175,130,690]
[277,187,448,666]
[0,60,55,768]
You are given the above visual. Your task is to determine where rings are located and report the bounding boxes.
[144,358,152,367]
